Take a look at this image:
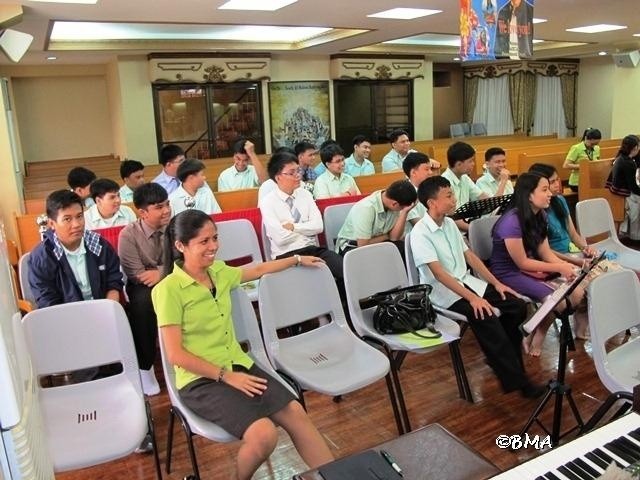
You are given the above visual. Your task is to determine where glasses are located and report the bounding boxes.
[275,168,302,177]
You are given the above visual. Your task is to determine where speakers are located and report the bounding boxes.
[0,28,33,63]
[612,50,640,67]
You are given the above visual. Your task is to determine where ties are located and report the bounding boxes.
[284,196,304,224]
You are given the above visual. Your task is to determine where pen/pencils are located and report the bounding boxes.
[380,449,404,478]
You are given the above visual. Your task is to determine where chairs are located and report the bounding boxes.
[10,119,640,480]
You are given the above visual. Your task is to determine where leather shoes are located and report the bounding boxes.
[522,382,549,400]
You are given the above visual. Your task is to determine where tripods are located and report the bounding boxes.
[508,298,588,453]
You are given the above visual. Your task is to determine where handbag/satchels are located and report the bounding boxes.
[371,282,444,341]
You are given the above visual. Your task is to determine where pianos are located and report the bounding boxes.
[487,409,640,480]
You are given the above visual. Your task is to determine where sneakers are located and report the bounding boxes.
[139,364,161,397]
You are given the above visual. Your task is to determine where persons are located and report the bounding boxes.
[529,162,610,342]
[152,209,336,480]
[561,126,603,192]
[28,190,154,453]
[486,170,582,358]
[608,136,640,243]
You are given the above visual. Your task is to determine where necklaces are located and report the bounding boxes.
[190,266,214,295]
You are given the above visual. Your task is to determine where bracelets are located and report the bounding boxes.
[218,364,227,384]
[294,252,302,267]
